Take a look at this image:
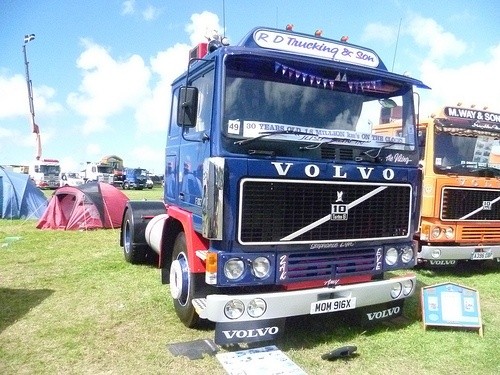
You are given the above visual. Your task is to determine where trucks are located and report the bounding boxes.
[9,159,61,190]
[120,24,432,345]
[360,93,500,267]
[72,160,114,184]
[107,169,148,191]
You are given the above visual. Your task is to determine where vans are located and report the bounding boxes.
[60,172,84,187]
[145,176,153,188]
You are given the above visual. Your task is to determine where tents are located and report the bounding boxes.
[1,165,48,222]
[35,183,130,229]
